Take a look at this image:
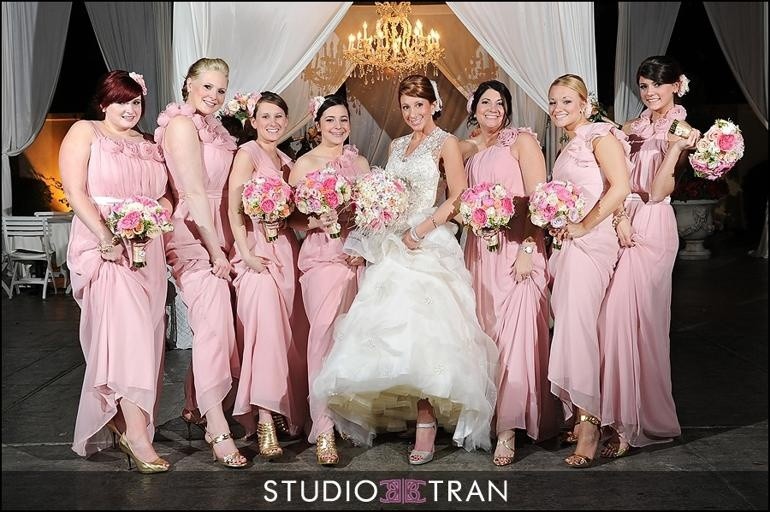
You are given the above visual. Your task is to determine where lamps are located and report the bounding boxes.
[340,3,448,87]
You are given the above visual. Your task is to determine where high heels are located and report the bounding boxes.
[106,408,247,474]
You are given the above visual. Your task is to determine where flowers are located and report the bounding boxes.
[668,115,747,183]
[239,167,588,254]
[670,173,730,201]
[100,194,176,271]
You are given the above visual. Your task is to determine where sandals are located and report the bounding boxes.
[565,414,630,468]
[257,411,339,464]
[409,422,437,464]
[492,429,515,465]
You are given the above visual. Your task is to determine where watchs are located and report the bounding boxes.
[519,246,533,254]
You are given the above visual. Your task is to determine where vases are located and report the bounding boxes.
[671,199,719,262]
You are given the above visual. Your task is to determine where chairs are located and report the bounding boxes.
[0,204,73,302]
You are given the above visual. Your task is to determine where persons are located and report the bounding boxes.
[313,74,500,466]
[287,96,373,467]
[58,69,171,472]
[457,79,547,467]
[596,54,700,460]
[154,58,248,468]
[549,73,632,466]
[227,92,309,460]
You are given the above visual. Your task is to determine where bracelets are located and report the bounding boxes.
[612,211,629,226]
[430,215,438,229]
[410,227,425,242]
